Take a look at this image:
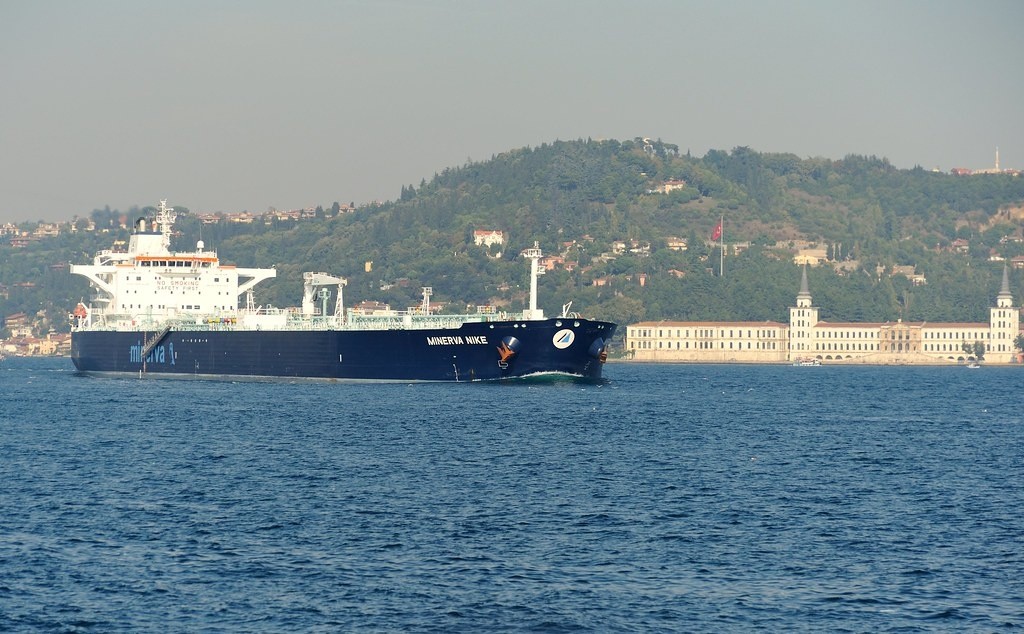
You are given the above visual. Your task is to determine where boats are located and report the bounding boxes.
[68,198,620,382]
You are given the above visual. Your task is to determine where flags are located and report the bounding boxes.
[712,223,722,241]
